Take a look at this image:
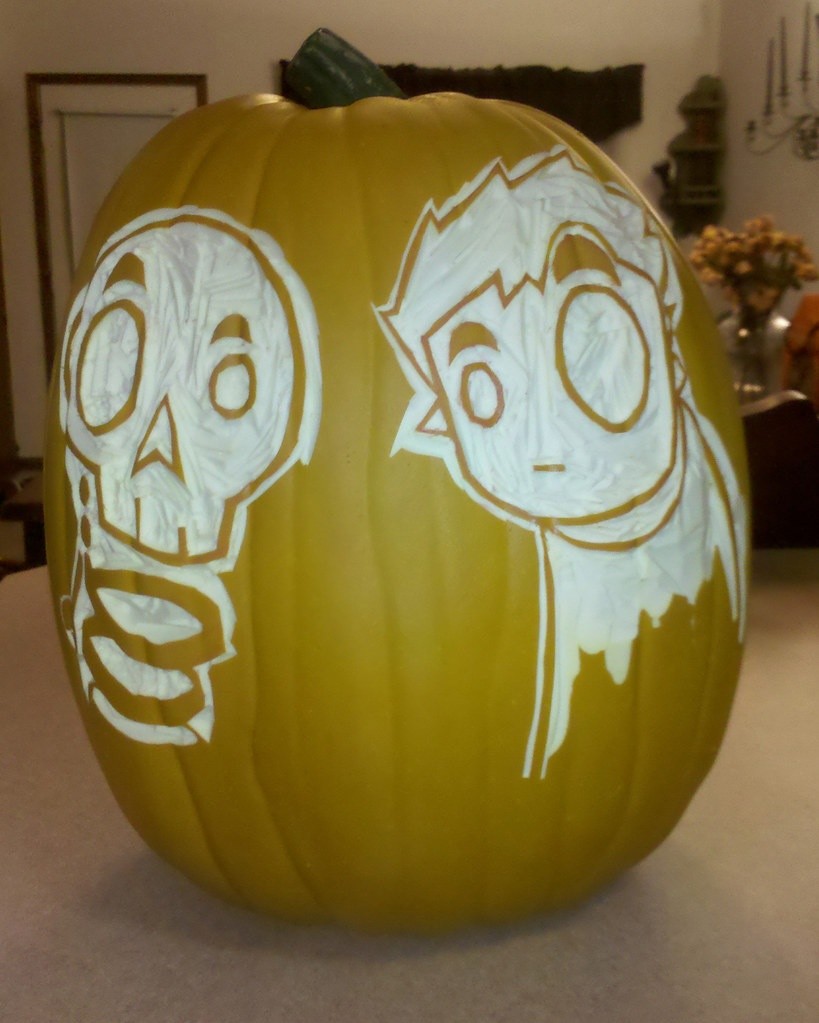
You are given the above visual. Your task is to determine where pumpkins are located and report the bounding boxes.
[42,26,751,936]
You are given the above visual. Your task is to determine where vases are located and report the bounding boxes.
[723,307,781,399]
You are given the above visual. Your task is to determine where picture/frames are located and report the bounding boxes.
[23,71,208,396]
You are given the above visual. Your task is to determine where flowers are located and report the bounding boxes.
[690,219,819,394]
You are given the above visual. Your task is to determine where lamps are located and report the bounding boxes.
[746,0,818,162]
